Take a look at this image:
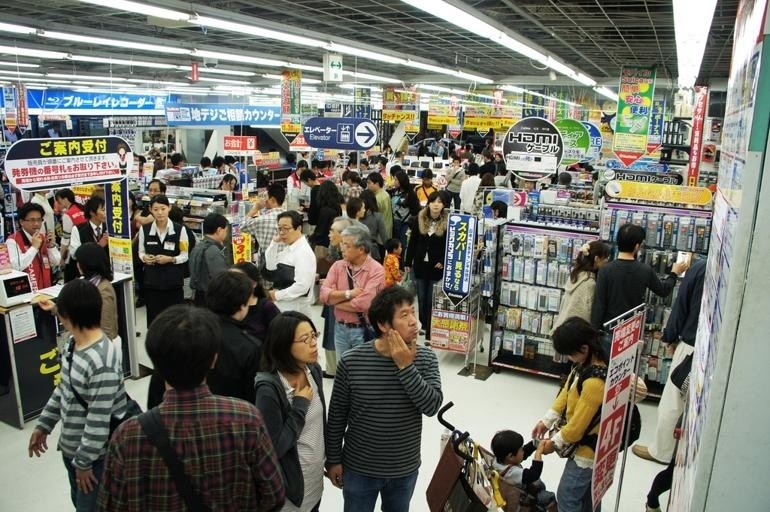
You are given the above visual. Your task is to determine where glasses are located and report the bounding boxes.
[276,225,296,233]
[19,216,44,224]
[294,330,321,344]
[338,242,358,249]
[147,187,161,194]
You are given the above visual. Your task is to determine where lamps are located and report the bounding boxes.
[670,0,719,87]
[396,0,622,102]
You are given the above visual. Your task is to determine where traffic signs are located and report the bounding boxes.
[304,116,378,148]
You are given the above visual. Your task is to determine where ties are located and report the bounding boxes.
[95,226,104,244]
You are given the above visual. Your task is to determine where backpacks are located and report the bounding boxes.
[585,400,643,458]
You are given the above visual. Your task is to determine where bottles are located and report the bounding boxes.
[192,173,227,189]
[229,199,254,221]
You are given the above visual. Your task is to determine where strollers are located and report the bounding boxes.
[426,401,560,512]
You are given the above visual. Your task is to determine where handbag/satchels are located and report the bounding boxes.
[395,279,418,301]
[104,400,148,447]
[364,324,378,341]
[271,263,314,299]
[548,420,578,459]
[438,429,500,512]
[671,350,694,391]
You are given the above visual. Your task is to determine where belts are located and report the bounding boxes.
[335,319,365,329]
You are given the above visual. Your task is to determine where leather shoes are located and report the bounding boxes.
[419,328,425,337]
[631,443,670,466]
[646,503,662,512]
[424,338,430,346]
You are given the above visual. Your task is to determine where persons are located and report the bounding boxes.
[325,285,441,511]
[254,311,328,512]
[647,352,695,511]
[2,129,598,379]
[490,431,558,512]
[531,315,609,512]
[149,269,261,410]
[631,252,706,465]
[94,306,286,511]
[549,223,688,400]
[29,280,127,511]
